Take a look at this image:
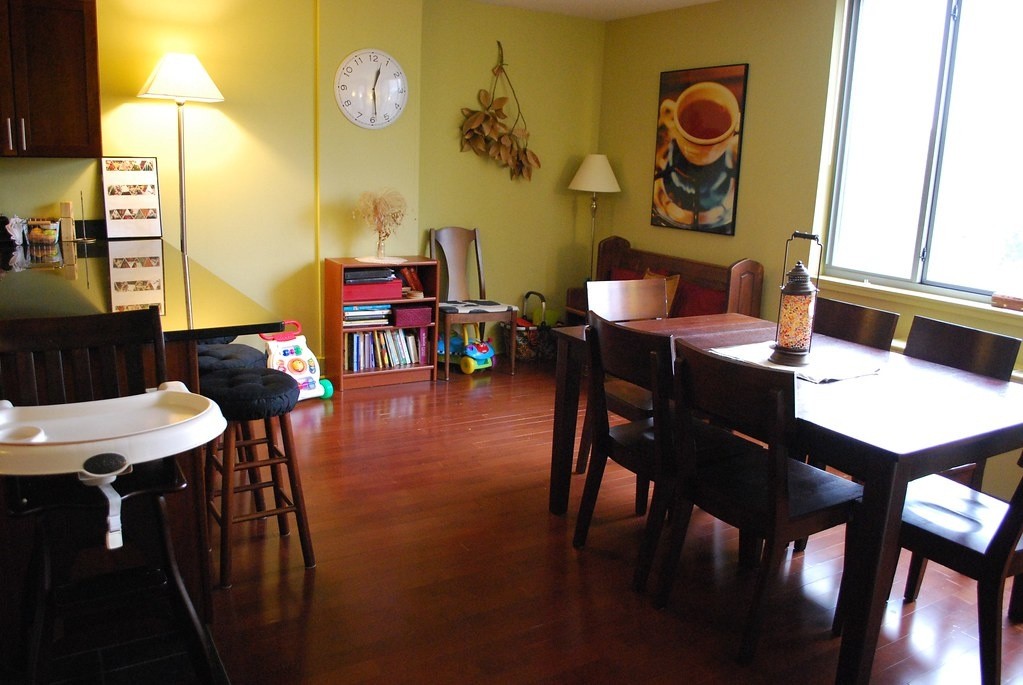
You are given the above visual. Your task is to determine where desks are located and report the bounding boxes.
[549,311,1023,685]
[0,233,285,659]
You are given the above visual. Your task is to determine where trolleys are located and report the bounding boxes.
[258,319,334,402]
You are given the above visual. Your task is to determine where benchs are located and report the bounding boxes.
[565,236,764,325]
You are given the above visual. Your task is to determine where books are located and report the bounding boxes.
[344,329,430,372]
[342,304,392,326]
[394,267,424,291]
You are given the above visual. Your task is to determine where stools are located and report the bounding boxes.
[198,344,317,589]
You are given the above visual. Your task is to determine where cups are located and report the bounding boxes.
[660,81,740,166]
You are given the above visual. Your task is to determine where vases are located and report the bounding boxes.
[377,231,385,258]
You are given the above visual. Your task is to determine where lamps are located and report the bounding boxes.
[769,232,822,368]
[137,52,225,254]
[567,154,621,280]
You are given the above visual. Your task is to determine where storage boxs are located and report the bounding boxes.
[343,278,402,303]
[59,201,77,241]
[390,303,432,327]
[405,328,429,365]
[991,292,1023,311]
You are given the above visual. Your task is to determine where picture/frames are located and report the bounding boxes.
[651,63,749,237]
[0,0,104,158]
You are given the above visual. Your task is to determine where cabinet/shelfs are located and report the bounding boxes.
[324,255,441,391]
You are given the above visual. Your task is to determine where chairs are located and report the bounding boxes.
[571,278,1023,685]
[1,303,234,685]
[430,226,520,381]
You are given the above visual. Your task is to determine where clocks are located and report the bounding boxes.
[334,47,408,129]
